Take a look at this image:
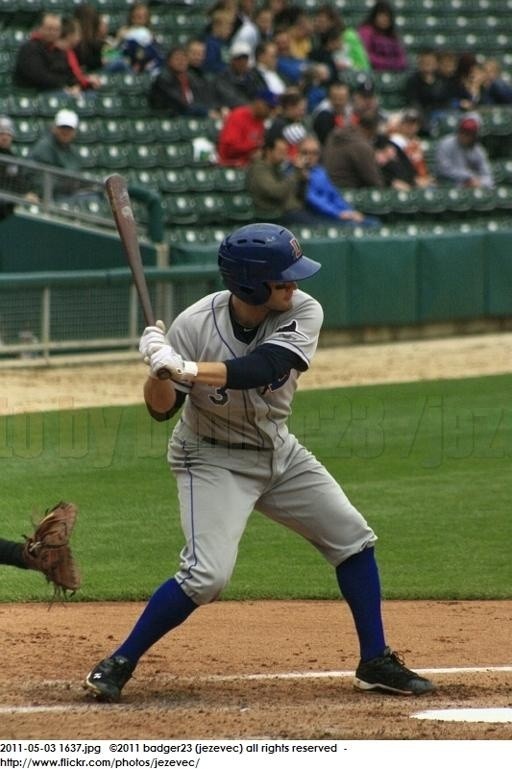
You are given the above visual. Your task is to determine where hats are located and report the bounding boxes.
[56,110,79,130]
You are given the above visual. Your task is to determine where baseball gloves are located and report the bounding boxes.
[22,501,79,589]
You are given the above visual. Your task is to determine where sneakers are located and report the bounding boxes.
[81,654,134,703]
[351,646,434,695]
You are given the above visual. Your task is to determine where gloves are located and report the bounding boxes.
[139,319,198,385]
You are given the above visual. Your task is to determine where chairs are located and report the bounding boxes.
[0,0,510,253]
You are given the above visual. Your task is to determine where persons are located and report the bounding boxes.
[0,499,82,616]
[1,1,511,222]
[81,220,433,702]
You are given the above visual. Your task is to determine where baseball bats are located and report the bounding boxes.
[103,171,172,380]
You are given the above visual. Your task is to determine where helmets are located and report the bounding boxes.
[218,223,322,306]
